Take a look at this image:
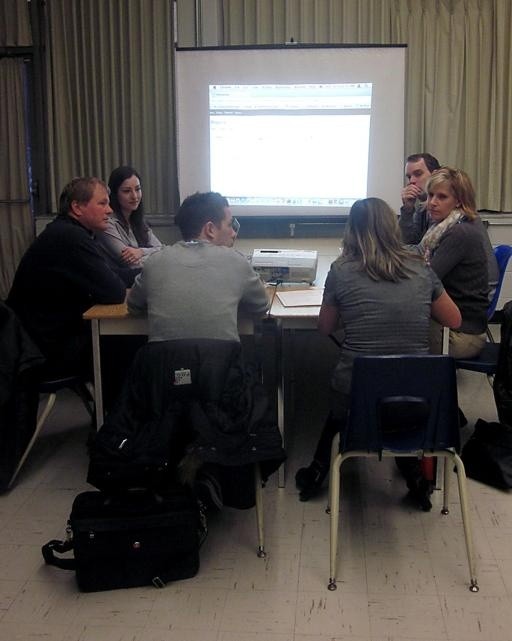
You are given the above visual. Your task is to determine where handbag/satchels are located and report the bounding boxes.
[39,487,209,596]
[451,417,511,493]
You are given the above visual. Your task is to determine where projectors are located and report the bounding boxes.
[251,248,318,285]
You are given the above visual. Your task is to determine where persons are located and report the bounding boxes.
[394,153,441,243]
[414,166,496,361]
[88,163,161,280]
[295,198,464,519]
[6,174,142,416]
[125,191,272,340]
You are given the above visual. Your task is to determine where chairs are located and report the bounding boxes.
[329,354,480,592]
[430,245,511,388]
[2,301,95,492]
[100,338,271,559]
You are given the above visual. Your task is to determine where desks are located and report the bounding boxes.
[84,290,273,480]
[269,284,450,488]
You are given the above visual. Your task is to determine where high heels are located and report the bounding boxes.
[405,489,432,512]
[295,467,324,503]
